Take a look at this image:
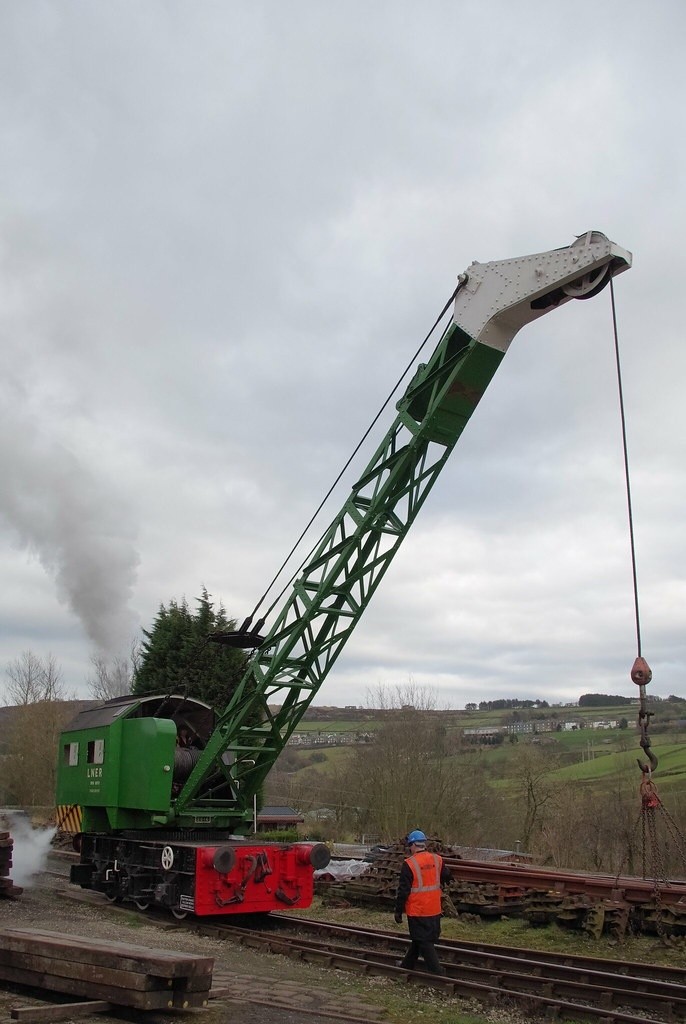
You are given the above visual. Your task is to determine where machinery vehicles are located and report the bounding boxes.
[51,231,662,922]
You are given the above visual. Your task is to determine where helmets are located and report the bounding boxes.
[407,830,426,846]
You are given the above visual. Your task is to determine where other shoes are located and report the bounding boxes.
[395,960,416,973]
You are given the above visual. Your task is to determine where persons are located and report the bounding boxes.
[175,725,198,750]
[393,830,457,977]
